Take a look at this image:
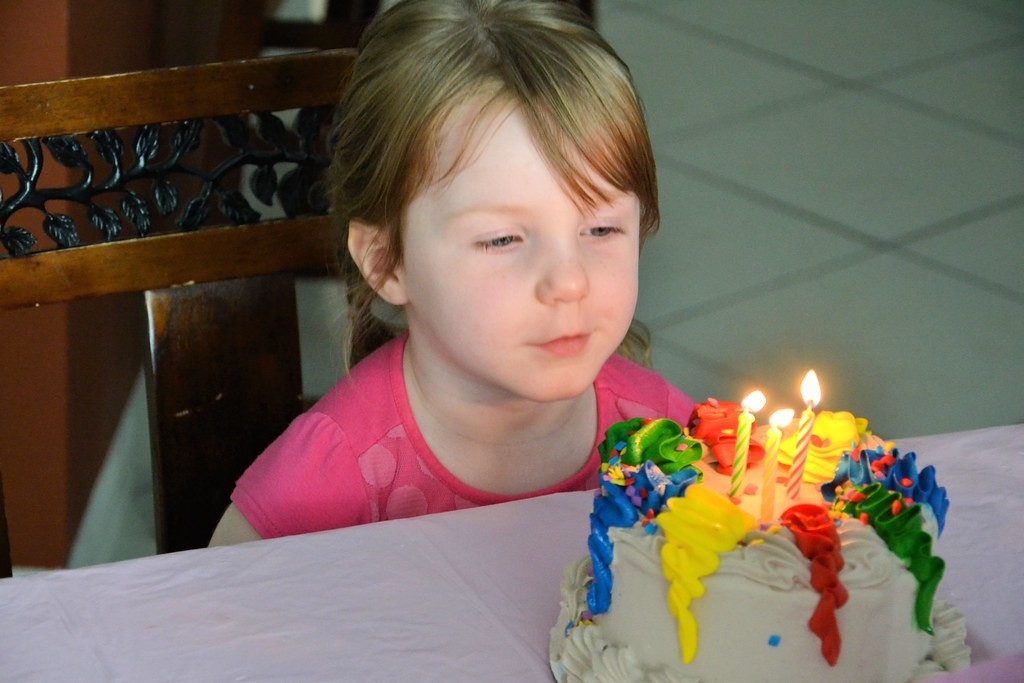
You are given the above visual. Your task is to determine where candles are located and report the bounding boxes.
[785,369,821,500]
[730,390,766,499]
[760,407,795,523]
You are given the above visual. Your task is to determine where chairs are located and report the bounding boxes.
[0,47,398,576]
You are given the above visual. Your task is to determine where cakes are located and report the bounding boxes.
[548,397,973,683]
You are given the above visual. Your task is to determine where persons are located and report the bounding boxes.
[205,1,702,547]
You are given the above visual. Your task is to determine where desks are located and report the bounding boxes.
[0,422,1024,683]
